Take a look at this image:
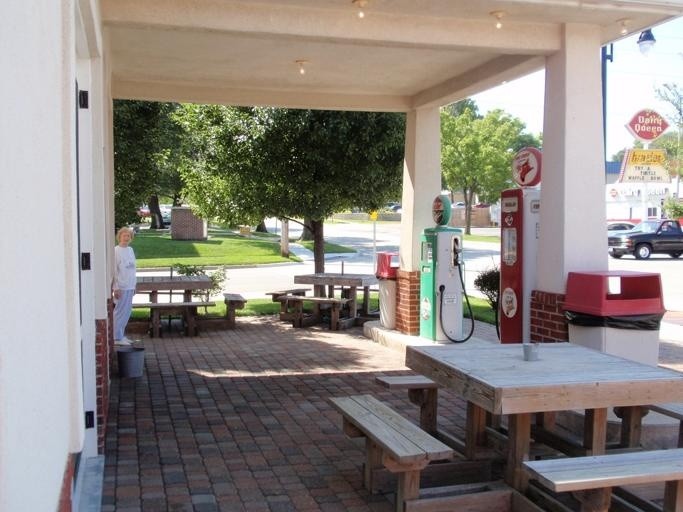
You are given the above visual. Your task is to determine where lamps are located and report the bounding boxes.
[353,0,369,18]
[617,19,631,37]
[296,59,311,74]
[491,11,508,29]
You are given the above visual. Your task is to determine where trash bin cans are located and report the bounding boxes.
[562,271,666,365]
[376,252,399,330]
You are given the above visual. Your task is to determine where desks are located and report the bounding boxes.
[405,342,683,490]
[137,275,213,337]
[294,272,380,329]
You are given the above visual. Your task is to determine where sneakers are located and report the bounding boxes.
[114,337,134,347]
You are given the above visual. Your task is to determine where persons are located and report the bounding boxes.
[112,226,137,345]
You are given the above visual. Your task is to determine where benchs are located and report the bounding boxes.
[520,445,682,512]
[328,393,455,512]
[130,292,246,337]
[376,375,555,438]
[266,287,382,330]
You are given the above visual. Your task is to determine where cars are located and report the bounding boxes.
[136,202,171,224]
[608,218,682,261]
[386,201,401,213]
[452,202,465,209]
[474,203,489,209]
